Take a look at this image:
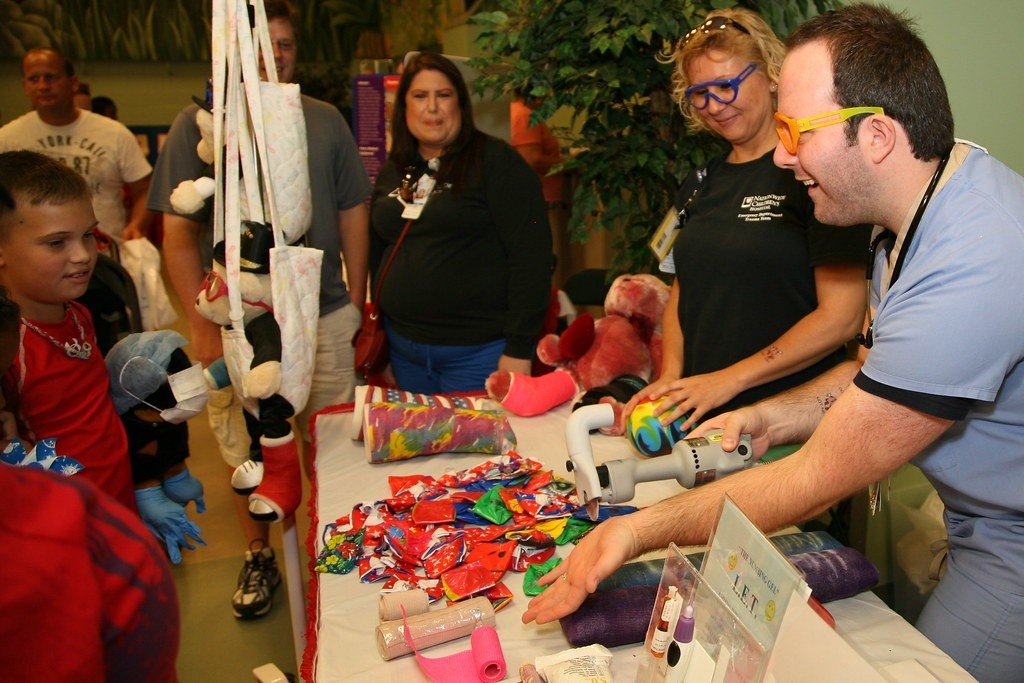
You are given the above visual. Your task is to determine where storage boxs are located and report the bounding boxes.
[351,74,400,208]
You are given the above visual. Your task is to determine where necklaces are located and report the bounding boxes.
[402,161,453,198]
[18,306,92,358]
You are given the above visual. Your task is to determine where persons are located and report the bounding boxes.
[510,73,566,290]
[371,53,555,395]
[0,47,181,683]
[521,6,1024,683]
[146,0,370,619]
[618,9,877,545]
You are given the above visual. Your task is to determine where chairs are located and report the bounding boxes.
[557,268,625,336]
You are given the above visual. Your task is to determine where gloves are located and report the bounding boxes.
[134,485,206,563]
[165,466,207,513]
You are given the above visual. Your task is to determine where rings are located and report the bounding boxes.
[561,572,567,582]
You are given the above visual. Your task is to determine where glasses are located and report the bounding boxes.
[679,15,748,51]
[686,64,757,110]
[774,105,884,154]
[272,39,298,52]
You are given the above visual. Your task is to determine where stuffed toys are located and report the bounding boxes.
[169,78,215,216]
[196,219,303,524]
[487,272,671,436]
[105,329,206,566]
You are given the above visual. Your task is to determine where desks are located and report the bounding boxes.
[297,392,978,683]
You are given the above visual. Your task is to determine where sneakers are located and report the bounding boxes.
[232,537,281,616]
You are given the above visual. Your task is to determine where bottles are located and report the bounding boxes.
[663,606,695,683]
[651,586,678,658]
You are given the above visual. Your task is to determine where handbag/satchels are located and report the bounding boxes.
[354,302,385,377]
[113,237,179,328]
